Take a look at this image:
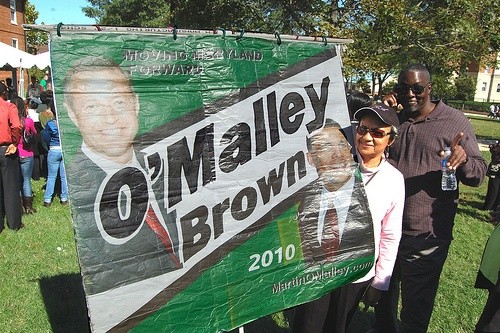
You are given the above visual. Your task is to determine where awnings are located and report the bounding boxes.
[0,41,52,70]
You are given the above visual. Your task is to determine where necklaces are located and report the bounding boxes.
[365,165,381,188]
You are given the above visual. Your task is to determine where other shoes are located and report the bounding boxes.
[43,201,51,206]
[60,200,68,205]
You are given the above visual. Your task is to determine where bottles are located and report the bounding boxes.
[440,147,457,190]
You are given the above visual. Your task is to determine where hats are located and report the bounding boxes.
[354,103,400,129]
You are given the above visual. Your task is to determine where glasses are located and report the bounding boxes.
[356,126,392,139]
[396,82,429,96]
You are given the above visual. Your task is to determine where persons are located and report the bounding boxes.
[297,118,375,271]
[0,70,69,231]
[488,104,500,121]
[375,64,487,333]
[63,56,184,295]
[346,88,384,121]
[292,102,405,333]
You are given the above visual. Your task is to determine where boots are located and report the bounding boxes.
[22,195,37,214]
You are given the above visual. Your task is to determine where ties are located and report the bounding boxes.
[144,204,181,270]
[321,194,339,262]
